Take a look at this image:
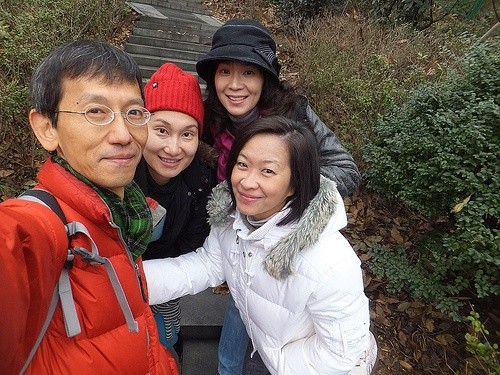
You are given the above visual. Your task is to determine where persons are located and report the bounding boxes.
[133,62,220,375]
[196,19,361,375]
[142,114,378,375]
[0,40,180,375]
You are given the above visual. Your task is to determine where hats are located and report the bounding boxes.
[196,19,282,88]
[144,63,204,140]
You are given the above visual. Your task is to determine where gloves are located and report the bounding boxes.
[150,297,181,343]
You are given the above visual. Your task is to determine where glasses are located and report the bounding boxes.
[42,104,155,126]
